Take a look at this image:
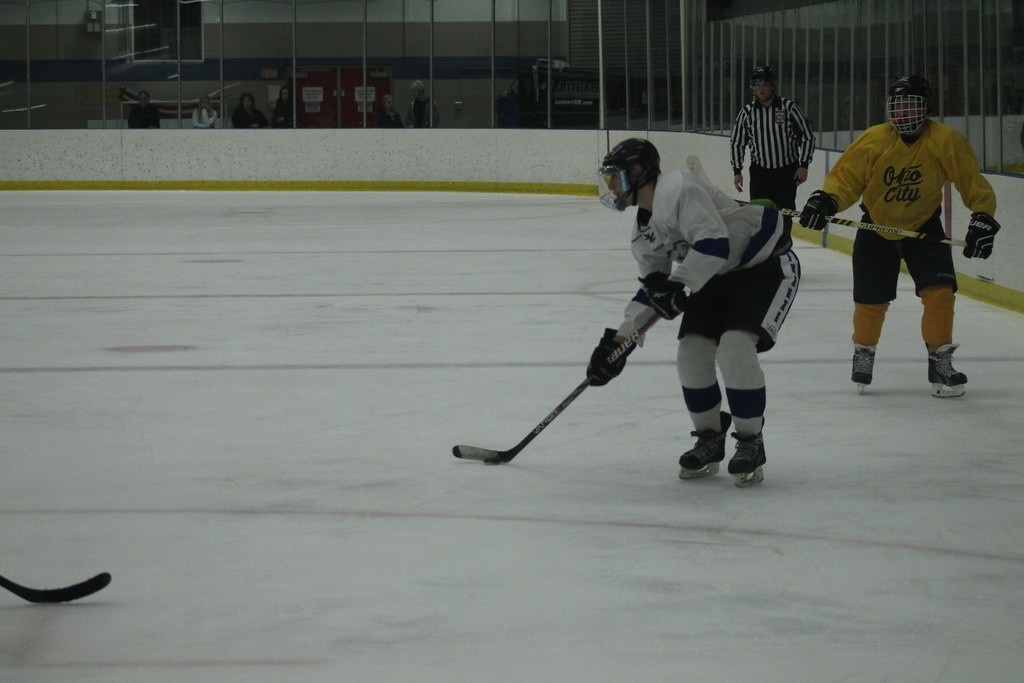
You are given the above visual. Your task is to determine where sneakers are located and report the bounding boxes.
[728,416,767,486]
[679,411,731,480]
[927,343,967,397]
[851,342,876,395]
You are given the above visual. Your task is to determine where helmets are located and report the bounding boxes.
[599,138,661,212]
[889,74,935,135]
[750,66,779,92]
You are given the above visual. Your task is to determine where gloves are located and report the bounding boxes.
[799,190,837,230]
[963,212,1001,259]
[586,328,636,386]
[643,271,695,321]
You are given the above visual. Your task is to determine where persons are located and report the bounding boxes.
[496,80,521,127]
[272,86,299,129]
[231,92,269,129]
[192,94,217,129]
[378,94,404,129]
[729,66,816,221]
[586,137,801,485]
[405,81,440,129]
[799,76,1002,398]
[128,90,160,130]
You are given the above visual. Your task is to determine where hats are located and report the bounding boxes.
[411,80,426,91]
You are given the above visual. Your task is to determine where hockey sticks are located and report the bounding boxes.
[449,310,665,465]
[0,572,112,604]
[685,153,965,246]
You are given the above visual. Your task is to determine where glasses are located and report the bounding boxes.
[385,101,395,104]
